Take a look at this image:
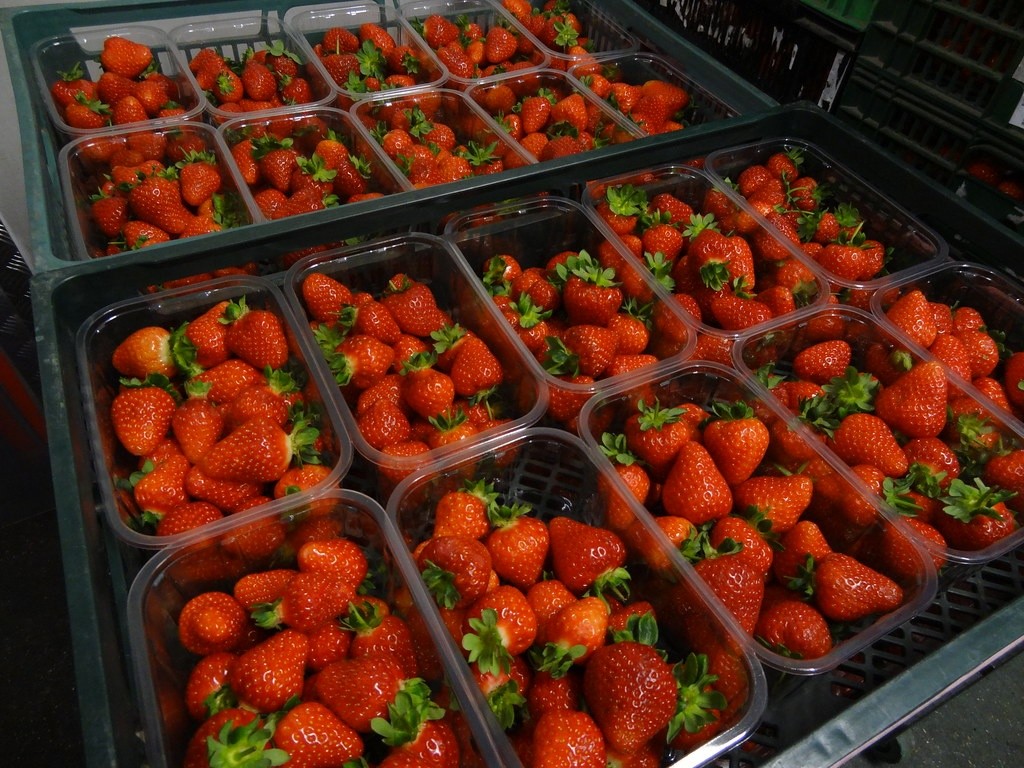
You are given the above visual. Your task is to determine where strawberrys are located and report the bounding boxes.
[51,0,1024,768]
